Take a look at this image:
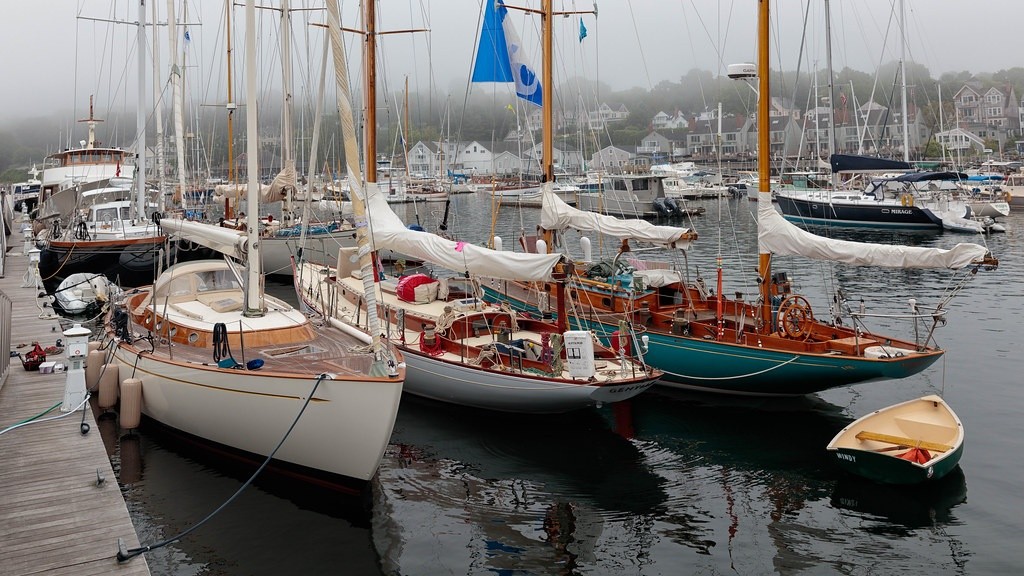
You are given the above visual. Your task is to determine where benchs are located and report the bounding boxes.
[855,431,952,453]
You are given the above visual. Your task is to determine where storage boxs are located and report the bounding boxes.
[863,346,917,358]
[39,361,57,374]
[53,363,65,374]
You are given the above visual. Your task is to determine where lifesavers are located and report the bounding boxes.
[1002,191,1012,202]
[902,193,913,206]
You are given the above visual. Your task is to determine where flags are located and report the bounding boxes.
[472,0,542,108]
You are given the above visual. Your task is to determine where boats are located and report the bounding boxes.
[6,5,1022,486]
